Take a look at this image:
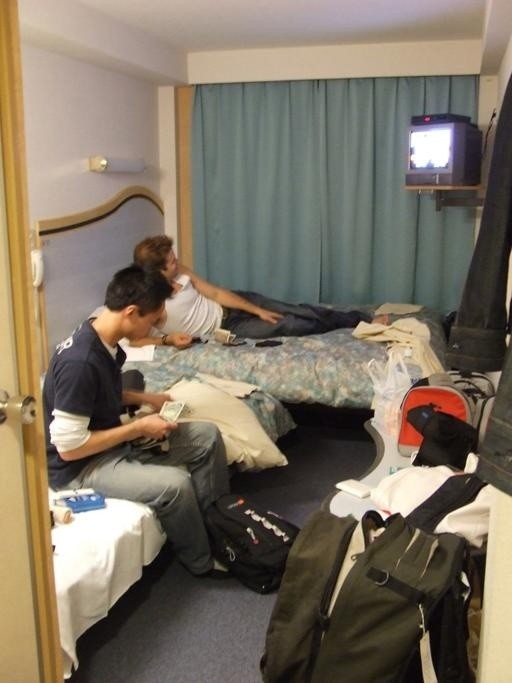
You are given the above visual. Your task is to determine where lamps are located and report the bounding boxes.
[89,152,145,175]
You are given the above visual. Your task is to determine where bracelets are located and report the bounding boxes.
[161,332,169,347]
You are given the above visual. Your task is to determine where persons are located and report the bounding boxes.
[128,236,390,348]
[43,263,239,580]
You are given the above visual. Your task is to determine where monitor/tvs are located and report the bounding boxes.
[405,122,482,186]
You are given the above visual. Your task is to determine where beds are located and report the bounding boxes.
[42,361,299,682]
[122,301,448,410]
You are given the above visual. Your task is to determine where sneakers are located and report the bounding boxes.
[190,560,232,578]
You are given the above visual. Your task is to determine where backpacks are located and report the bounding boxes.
[259,473,489,683]
[396,368,496,467]
[203,492,301,595]
